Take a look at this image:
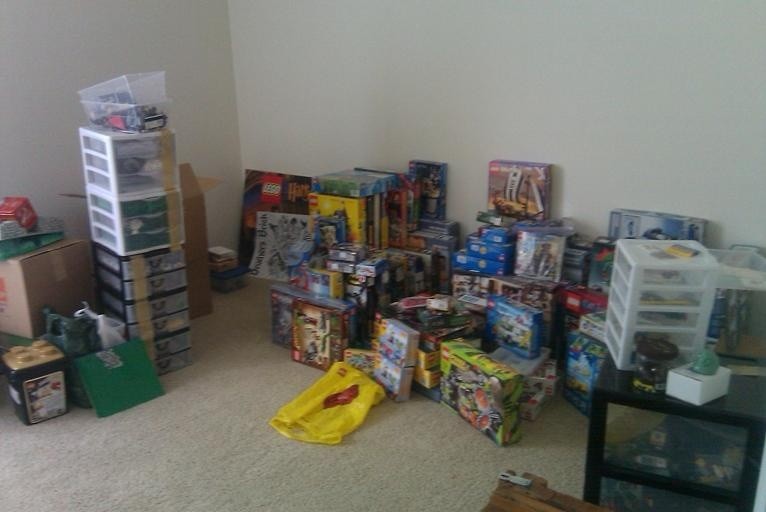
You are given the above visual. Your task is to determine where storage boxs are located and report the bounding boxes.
[0,70,251,426]
[243,160,765,449]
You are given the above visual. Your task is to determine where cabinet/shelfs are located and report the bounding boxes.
[483,355,765,512]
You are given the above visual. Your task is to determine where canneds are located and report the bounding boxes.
[631,340,679,395]
